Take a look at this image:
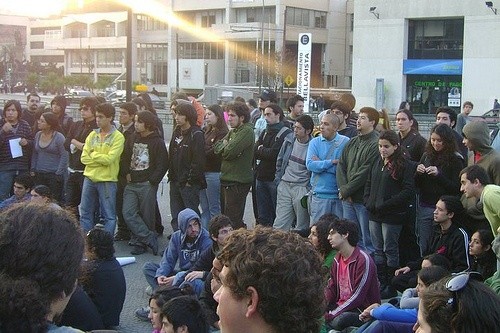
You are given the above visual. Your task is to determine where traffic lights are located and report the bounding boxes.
[7,63,13,73]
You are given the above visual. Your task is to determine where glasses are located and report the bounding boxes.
[79,107,88,110]
[446,270,483,293]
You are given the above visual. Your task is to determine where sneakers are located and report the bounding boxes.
[136,306,151,321]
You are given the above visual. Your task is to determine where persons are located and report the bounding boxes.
[415,273,500,333]
[0,93,168,255]
[460,165,500,293]
[308,220,338,286]
[168,88,427,264]
[175,214,234,295]
[390,196,470,293]
[322,217,381,333]
[362,130,414,267]
[455,101,473,138]
[213,224,326,333]
[460,116,500,227]
[494,99,500,116]
[148,284,193,333]
[161,295,208,333]
[355,265,451,333]
[0,195,127,333]
[355,254,451,333]
[469,227,497,282]
[415,123,464,256]
[136,208,212,321]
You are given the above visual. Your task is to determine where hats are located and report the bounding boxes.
[257,89,277,101]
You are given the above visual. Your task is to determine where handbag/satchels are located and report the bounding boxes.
[306,190,313,216]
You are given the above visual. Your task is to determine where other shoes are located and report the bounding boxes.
[114,231,131,241]
[148,233,159,256]
[95,220,105,227]
[130,244,148,255]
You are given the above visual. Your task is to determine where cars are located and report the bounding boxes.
[65,90,95,103]
[468,108,500,128]
[105,88,165,109]
